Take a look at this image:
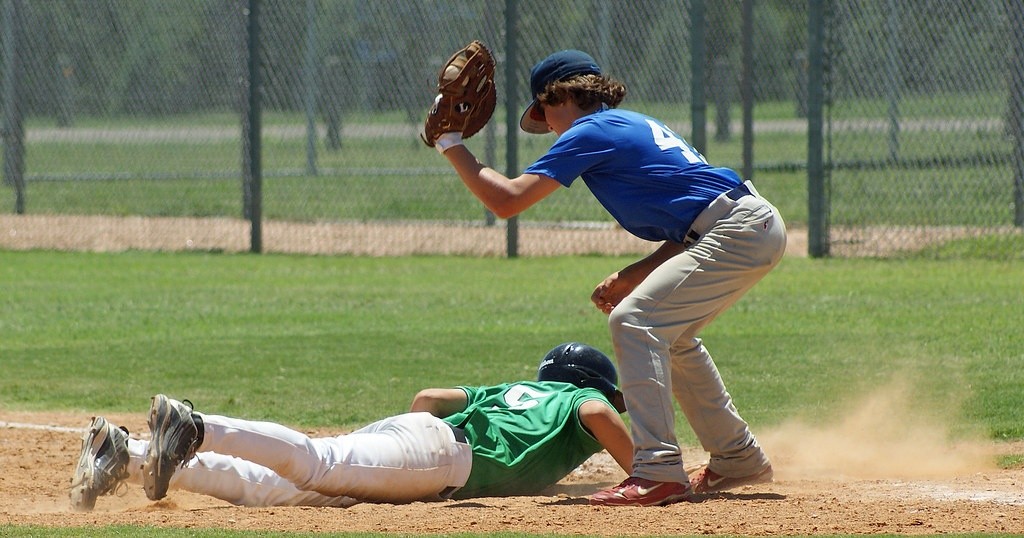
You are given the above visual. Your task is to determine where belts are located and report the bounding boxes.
[683,185,750,250]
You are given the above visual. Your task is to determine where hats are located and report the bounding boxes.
[520,49,600,135]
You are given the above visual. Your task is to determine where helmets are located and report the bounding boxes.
[539,342,627,413]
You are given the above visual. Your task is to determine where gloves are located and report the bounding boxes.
[432,94,469,154]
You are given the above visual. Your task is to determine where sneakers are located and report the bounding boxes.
[589,476,689,507]
[69,416,131,513]
[689,465,774,495]
[139,393,198,501]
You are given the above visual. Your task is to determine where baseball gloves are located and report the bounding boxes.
[420,40,498,149]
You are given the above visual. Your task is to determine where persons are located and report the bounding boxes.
[67,338,635,515]
[423,48,788,509]
